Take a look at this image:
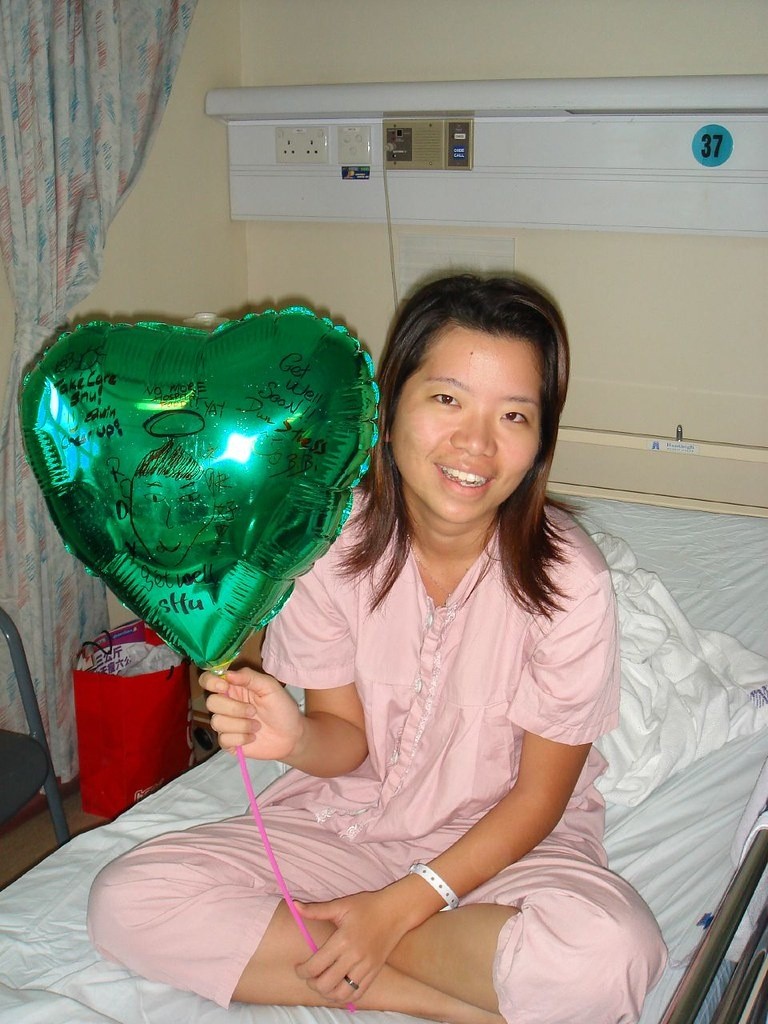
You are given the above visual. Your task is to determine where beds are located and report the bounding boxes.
[0,490,767,1024]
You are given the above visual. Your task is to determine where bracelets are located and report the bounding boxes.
[406,859,461,914]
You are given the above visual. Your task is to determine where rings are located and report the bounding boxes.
[344,976,360,992]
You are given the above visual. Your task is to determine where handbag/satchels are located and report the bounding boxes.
[72,619,195,822]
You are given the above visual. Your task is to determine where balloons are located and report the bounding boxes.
[17,305,374,671]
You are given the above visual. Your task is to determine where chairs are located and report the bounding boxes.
[0,607,70,849]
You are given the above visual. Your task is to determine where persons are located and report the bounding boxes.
[86,272,668,1024]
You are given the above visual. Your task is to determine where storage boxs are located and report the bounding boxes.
[70,618,196,821]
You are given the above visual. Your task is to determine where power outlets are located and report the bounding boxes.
[337,125,373,166]
[275,127,329,164]
